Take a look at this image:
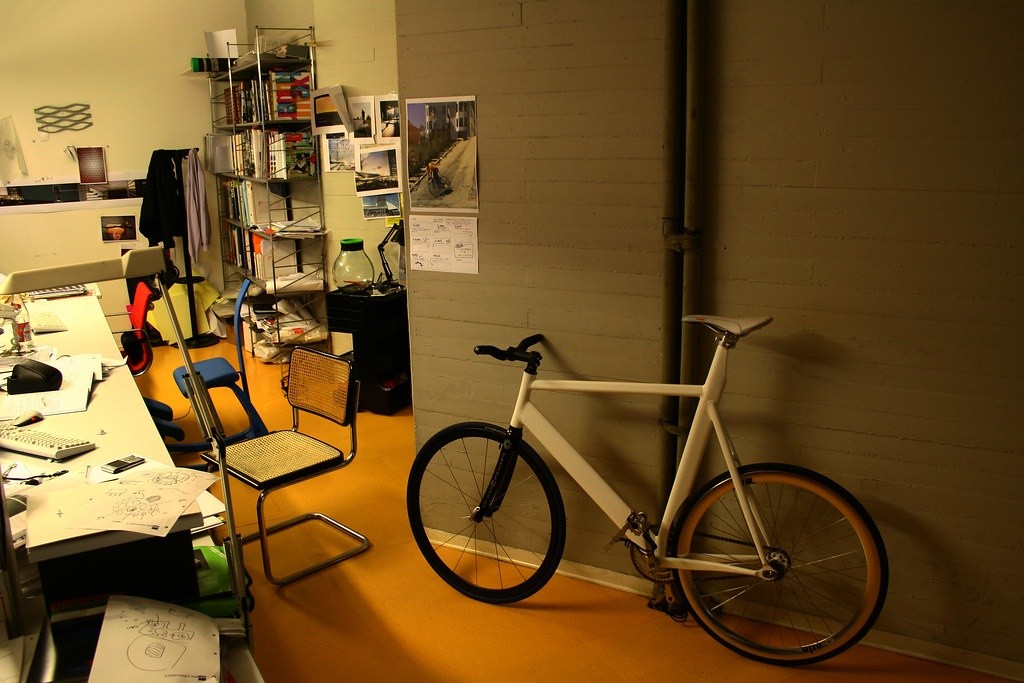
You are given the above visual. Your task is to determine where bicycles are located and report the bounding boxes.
[405,314,890,668]
[427,176,451,197]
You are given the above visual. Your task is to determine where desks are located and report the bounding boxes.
[0,282,203,683]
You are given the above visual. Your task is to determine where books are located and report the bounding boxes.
[210,74,322,284]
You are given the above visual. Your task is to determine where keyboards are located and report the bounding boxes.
[0,424,95,459]
[20,302,68,334]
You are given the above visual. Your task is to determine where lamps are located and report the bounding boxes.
[0,245,251,644]
[377,218,405,292]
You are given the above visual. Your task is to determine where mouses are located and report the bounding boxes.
[10,411,44,428]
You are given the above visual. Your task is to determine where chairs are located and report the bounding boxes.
[104,282,154,376]
[201,343,368,586]
[145,279,270,453]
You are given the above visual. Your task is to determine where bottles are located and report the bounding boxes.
[14,304,33,352]
[333,238,373,293]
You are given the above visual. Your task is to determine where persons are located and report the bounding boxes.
[424,160,444,187]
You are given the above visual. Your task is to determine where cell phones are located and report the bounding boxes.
[101,455,145,473]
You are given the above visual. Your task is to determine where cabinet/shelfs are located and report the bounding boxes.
[209,27,330,361]
[327,279,412,416]
